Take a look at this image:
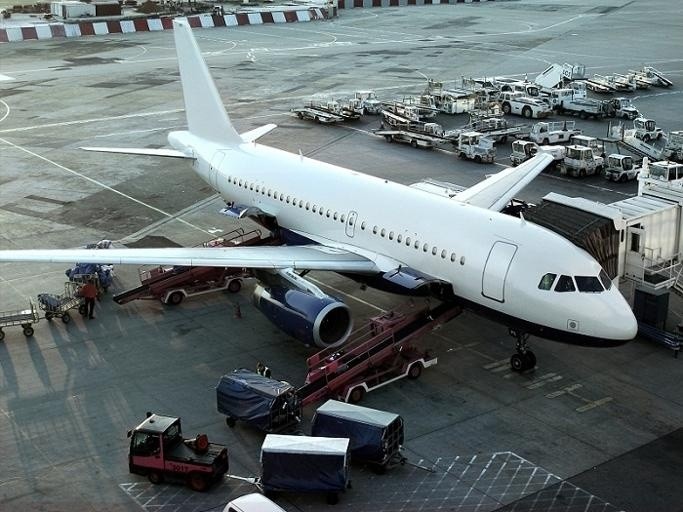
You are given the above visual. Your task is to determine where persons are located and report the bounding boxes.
[256,362,271,377]
[80,278,97,319]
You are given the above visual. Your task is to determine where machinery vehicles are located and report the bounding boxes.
[124,412,230,493]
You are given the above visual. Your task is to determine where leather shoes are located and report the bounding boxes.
[89,316,96,320]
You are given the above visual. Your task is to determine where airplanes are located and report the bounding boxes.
[2,19,635,377]
